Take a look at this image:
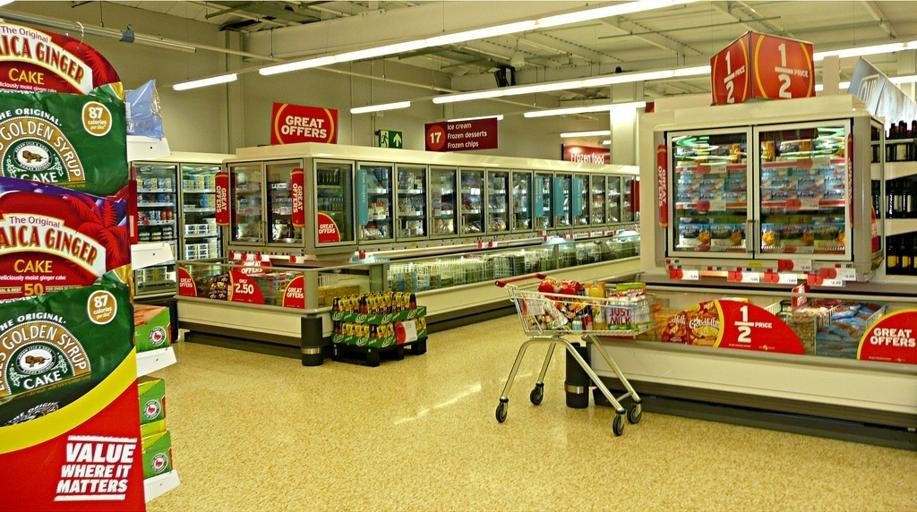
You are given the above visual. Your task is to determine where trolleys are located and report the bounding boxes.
[496,273,659,436]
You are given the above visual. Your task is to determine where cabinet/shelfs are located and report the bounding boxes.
[577,95,916,283]
[579,270,917,436]
[222,144,644,252]
[129,135,229,306]
[172,257,368,361]
[363,251,644,330]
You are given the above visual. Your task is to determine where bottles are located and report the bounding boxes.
[872,120,916,274]
[572,281,605,331]
[317,167,344,211]
[332,292,416,316]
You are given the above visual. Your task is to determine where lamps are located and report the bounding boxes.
[173,1,917,145]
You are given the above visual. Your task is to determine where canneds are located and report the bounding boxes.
[571,315,593,330]
[149,209,173,220]
